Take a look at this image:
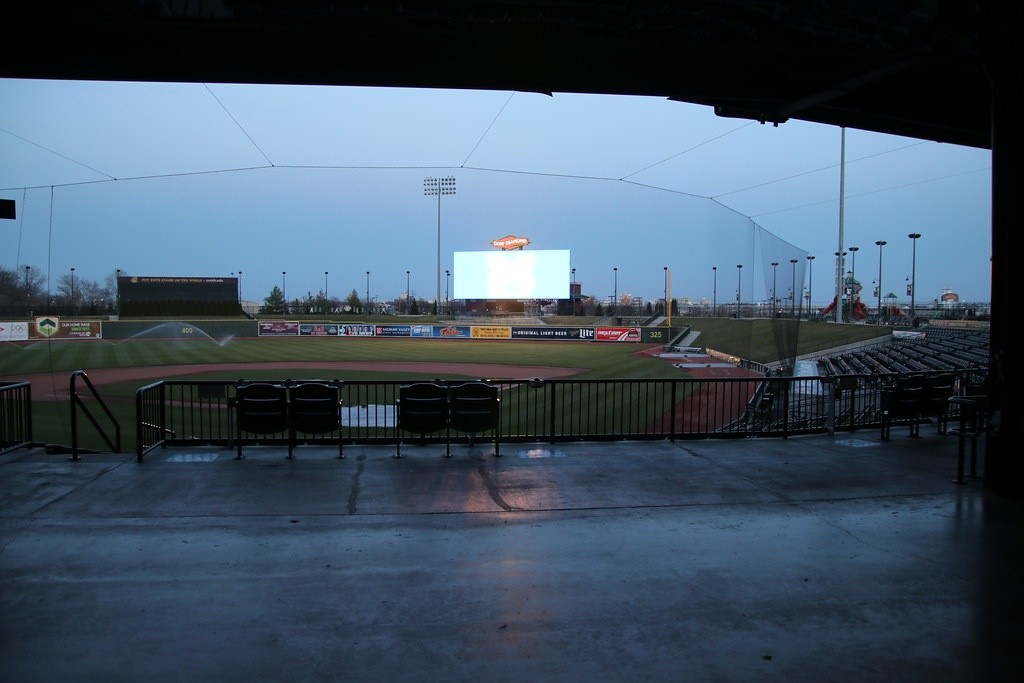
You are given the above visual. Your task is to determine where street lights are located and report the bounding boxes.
[664,266,667,317]
[366,271,370,303]
[70,267,75,299]
[806,255,815,315]
[789,259,798,316]
[116,269,121,298]
[282,271,286,301]
[875,240,888,325]
[736,264,743,318]
[848,247,859,323]
[712,266,717,315]
[324,271,328,300]
[446,270,450,312]
[908,233,921,320]
[771,262,779,308]
[614,268,617,306]
[238,271,242,305]
[572,268,577,316]
[406,270,410,304]
[834,251,847,297]
[423,175,457,315]
[26,266,31,285]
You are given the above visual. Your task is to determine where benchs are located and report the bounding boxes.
[880,373,955,441]
[396,383,500,458]
[236,383,345,460]
